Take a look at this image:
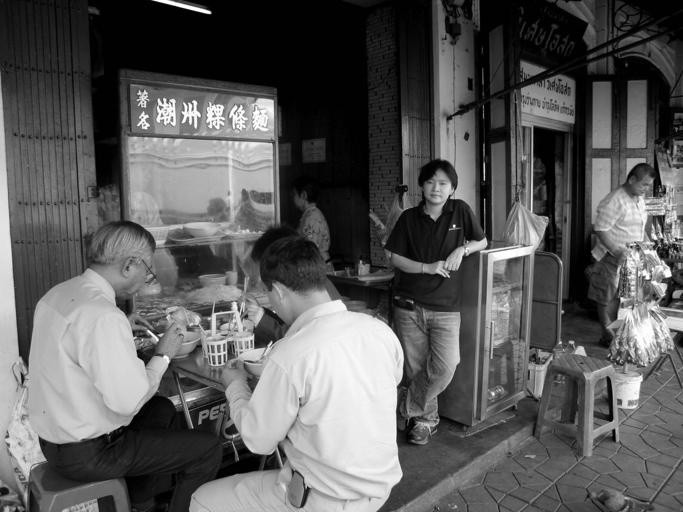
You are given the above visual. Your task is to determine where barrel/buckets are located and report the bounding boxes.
[196,273,227,285]
[616,369,643,410]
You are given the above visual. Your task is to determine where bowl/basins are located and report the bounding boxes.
[183,221,222,238]
[149,330,203,360]
[237,347,271,379]
[198,273,227,287]
[218,319,254,334]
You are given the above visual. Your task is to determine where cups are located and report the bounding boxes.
[225,271,238,285]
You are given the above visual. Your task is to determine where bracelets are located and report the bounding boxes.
[152,353,170,365]
[421,263,424,274]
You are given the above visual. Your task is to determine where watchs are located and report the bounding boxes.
[461,246,469,257]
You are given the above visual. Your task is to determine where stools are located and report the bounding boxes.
[533,355,620,457]
[23,461,131,511]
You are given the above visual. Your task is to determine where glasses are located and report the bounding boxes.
[141,260,157,283]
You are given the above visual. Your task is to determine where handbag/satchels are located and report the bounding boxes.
[5,355,100,511]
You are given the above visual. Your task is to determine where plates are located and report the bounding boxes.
[168,233,226,245]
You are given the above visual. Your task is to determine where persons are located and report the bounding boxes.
[382,158,488,446]
[187,235,407,511]
[586,163,657,348]
[291,174,334,274]
[235,222,343,347]
[26,220,225,511]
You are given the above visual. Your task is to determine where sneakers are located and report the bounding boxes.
[408,420,438,446]
[396,386,410,431]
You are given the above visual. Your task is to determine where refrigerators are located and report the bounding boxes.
[438,239,537,427]
